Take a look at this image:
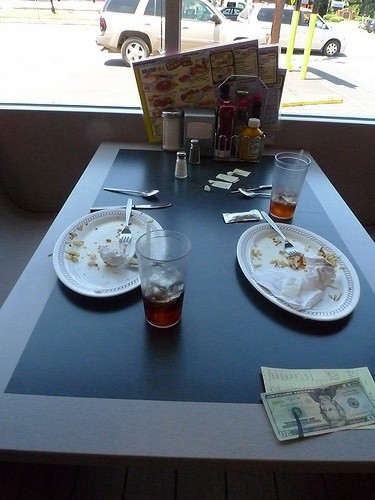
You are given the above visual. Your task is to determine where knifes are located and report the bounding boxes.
[231,184,272,193]
[90,203,171,212]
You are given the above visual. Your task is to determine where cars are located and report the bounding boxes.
[221,6,245,24]
[236,3,343,58]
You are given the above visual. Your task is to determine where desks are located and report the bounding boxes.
[0,141,375,500]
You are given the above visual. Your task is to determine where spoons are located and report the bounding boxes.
[102,188,160,197]
[238,188,271,197]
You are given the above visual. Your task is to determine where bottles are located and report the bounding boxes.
[161,109,184,152]
[216,83,266,161]
[188,139,200,164]
[175,151,187,179]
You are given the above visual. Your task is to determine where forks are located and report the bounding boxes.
[261,211,304,258]
[118,198,132,243]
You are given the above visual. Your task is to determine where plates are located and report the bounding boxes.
[52,208,166,297]
[237,222,361,322]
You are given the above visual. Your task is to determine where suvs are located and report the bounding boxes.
[96,0,272,67]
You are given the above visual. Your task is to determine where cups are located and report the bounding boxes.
[135,230,191,329]
[269,152,311,220]
